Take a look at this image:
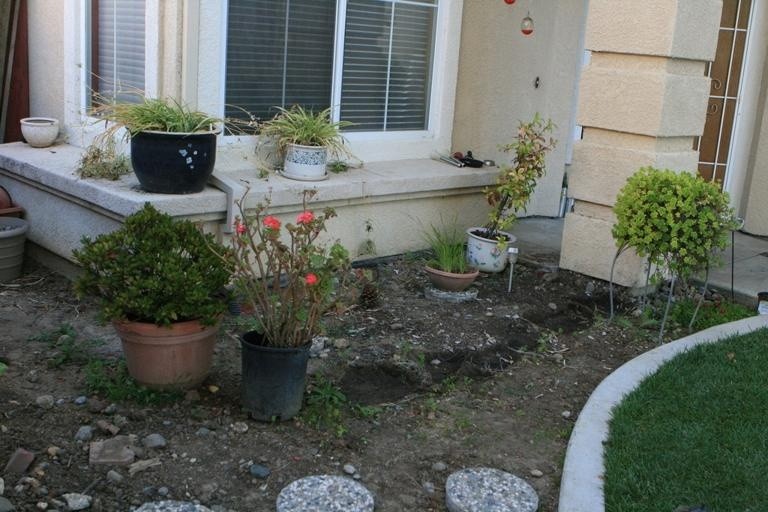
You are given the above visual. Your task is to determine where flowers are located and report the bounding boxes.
[191,184,354,347]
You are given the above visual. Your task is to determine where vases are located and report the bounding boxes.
[0,217,28,280]
[239,330,314,423]
[19,116,60,148]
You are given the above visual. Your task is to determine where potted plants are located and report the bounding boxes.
[252,101,365,195]
[415,205,480,291]
[68,201,240,395]
[466,111,560,274]
[67,63,264,194]
